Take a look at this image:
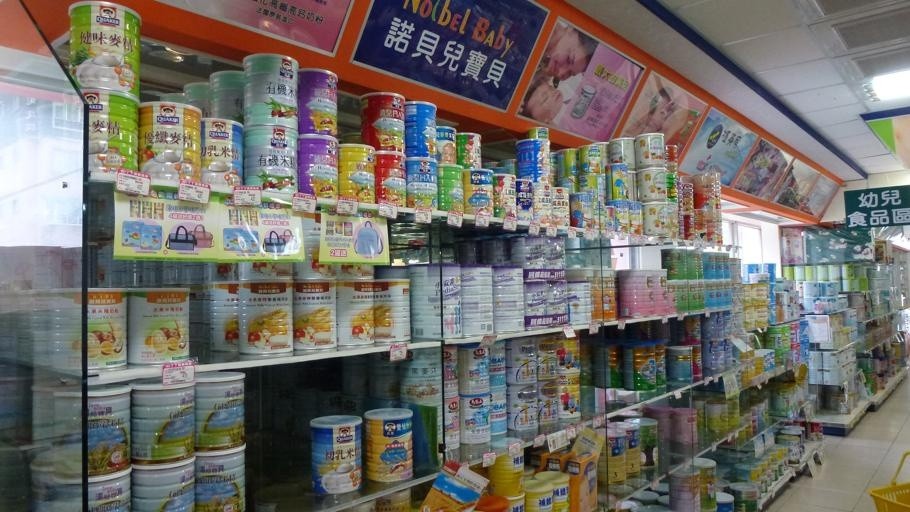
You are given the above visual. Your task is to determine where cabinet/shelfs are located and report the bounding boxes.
[736,274,827,512]
[774,245,909,432]
[1,165,734,509]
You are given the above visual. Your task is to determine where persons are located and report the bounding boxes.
[523,24,599,122]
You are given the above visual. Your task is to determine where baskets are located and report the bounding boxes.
[867,451,910,512]
